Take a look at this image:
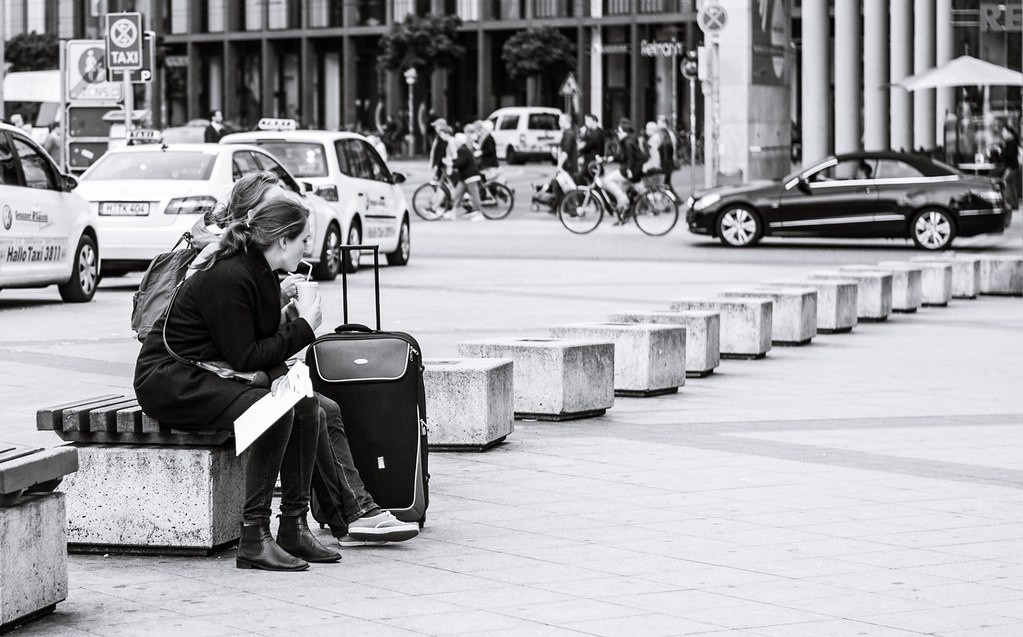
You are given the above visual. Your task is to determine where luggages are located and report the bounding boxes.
[305,244,429,528]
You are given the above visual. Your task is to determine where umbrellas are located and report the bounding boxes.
[878,55,1023,169]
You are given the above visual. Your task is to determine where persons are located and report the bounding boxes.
[427,109,438,146]
[990,126,1020,209]
[816,163,873,181]
[189,171,419,546]
[549,113,683,226]
[134,195,342,571]
[383,111,410,158]
[44,121,60,165]
[204,109,229,143]
[429,118,500,221]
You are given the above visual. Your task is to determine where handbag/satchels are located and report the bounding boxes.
[198,360,269,387]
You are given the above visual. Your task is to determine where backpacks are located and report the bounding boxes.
[131,230,203,342]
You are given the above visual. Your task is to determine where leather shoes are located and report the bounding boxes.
[236,521,310,572]
[276,512,342,561]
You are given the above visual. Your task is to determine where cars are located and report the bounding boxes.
[160,126,206,144]
[72,144,344,282]
[686,150,1012,252]
[0,120,103,304]
[219,119,411,275]
[485,107,563,165]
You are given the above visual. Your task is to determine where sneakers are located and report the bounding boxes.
[348,510,420,542]
[339,533,391,547]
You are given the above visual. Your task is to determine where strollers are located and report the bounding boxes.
[531,169,559,212]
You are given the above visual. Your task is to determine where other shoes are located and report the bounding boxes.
[468,215,483,223]
[443,210,457,221]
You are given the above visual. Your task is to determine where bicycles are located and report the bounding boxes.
[412,161,515,220]
[559,154,679,237]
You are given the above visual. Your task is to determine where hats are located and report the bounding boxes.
[481,120,493,132]
[430,118,446,126]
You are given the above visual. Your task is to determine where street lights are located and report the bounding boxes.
[404,68,418,160]
